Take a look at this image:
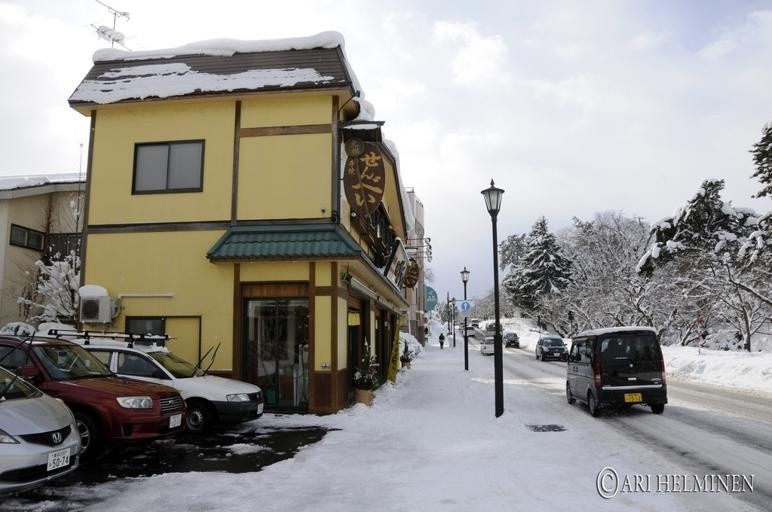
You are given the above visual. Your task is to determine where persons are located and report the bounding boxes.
[439,333,445,349]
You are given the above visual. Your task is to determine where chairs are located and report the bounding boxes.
[121,358,147,376]
[603,340,621,356]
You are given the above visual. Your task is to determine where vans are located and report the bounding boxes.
[566,325,668,417]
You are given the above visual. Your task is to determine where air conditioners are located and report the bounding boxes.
[79,298,112,326]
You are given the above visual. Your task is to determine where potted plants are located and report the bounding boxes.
[354,346,378,403]
[400,340,413,368]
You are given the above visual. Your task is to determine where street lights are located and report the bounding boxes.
[451,178,506,417]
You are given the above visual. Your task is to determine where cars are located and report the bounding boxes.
[536,337,569,362]
[459,318,519,355]
